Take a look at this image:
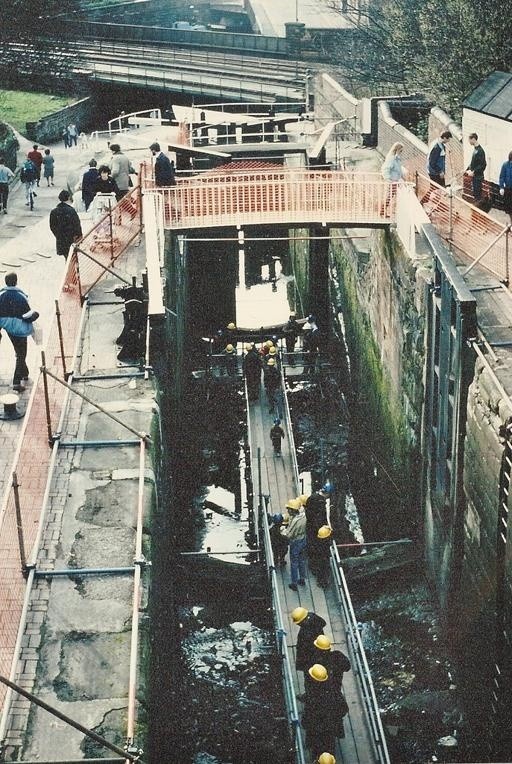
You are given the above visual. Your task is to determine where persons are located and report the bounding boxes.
[82,159,101,211]
[270,417,285,458]
[379,142,406,217]
[313,635,351,688]
[221,312,317,411]
[315,750,336,763]
[300,664,349,759]
[498,152,512,215]
[92,166,120,237]
[42,148,54,185]
[268,106,277,117]
[49,190,83,293]
[149,142,180,219]
[20,158,37,205]
[118,111,127,128]
[419,132,454,206]
[28,145,43,186]
[272,480,334,590]
[63,127,71,148]
[68,124,77,147]
[79,132,88,149]
[467,133,486,205]
[0,272,38,391]
[0,159,15,215]
[292,606,327,701]
[109,143,138,225]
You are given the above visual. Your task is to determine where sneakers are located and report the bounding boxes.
[286,579,307,592]
[12,373,29,391]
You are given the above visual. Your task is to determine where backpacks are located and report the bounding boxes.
[23,160,35,175]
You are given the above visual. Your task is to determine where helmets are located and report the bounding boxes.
[269,494,310,525]
[273,418,282,424]
[225,343,234,353]
[288,311,298,318]
[316,752,336,764]
[307,663,330,682]
[313,634,331,651]
[246,344,252,352]
[290,606,309,625]
[264,340,277,366]
[226,322,236,330]
[320,483,336,496]
[316,525,332,539]
